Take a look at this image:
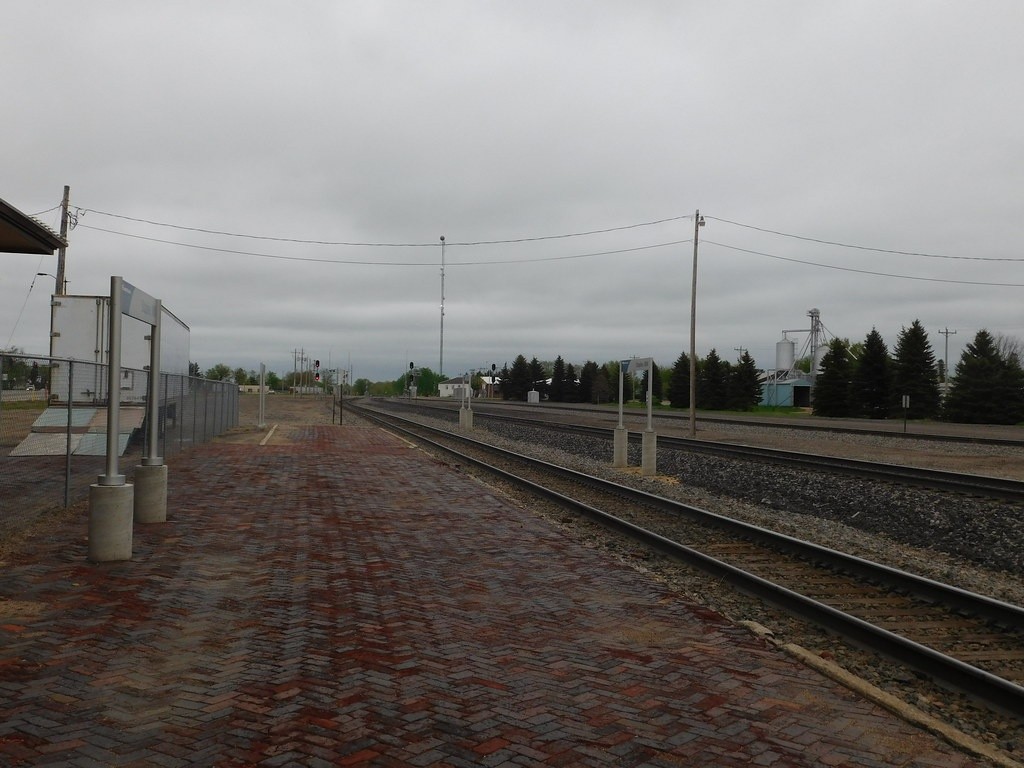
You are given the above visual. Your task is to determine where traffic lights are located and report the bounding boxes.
[315,359,319,369]
[315,372,319,381]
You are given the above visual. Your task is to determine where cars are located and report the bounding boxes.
[268,389,275,395]
[26,384,36,391]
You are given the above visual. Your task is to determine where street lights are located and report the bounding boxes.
[689,209,706,436]
[440,235,448,378]
